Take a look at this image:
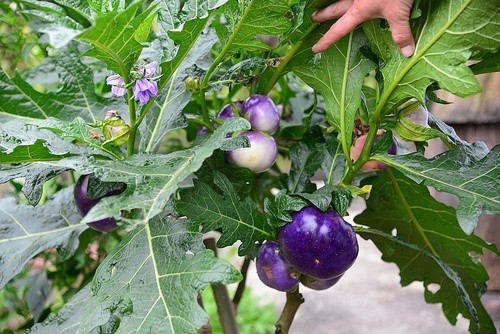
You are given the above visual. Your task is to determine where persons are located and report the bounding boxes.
[310,0,420,59]
